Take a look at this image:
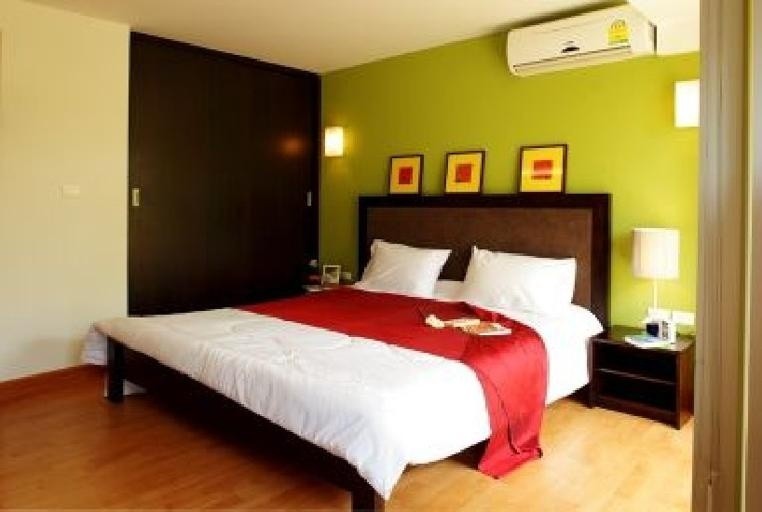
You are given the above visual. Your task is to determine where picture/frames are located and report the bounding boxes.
[443,150,485,195]
[517,143,567,194]
[387,153,425,197]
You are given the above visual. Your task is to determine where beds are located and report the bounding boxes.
[106,193,612,512]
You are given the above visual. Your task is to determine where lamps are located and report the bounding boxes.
[325,125,344,158]
[632,228,681,335]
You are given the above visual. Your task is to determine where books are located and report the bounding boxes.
[625,335,671,350]
[304,284,333,292]
[462,323,512,335]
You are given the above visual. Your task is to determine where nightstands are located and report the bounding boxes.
[587,324,696,431]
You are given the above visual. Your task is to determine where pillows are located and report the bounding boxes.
[351,237,453,299]
[459,245,578,324]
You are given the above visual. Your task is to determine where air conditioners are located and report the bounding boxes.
[506,4,656,78]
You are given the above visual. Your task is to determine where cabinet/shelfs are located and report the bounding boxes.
[129,32,321,318]
